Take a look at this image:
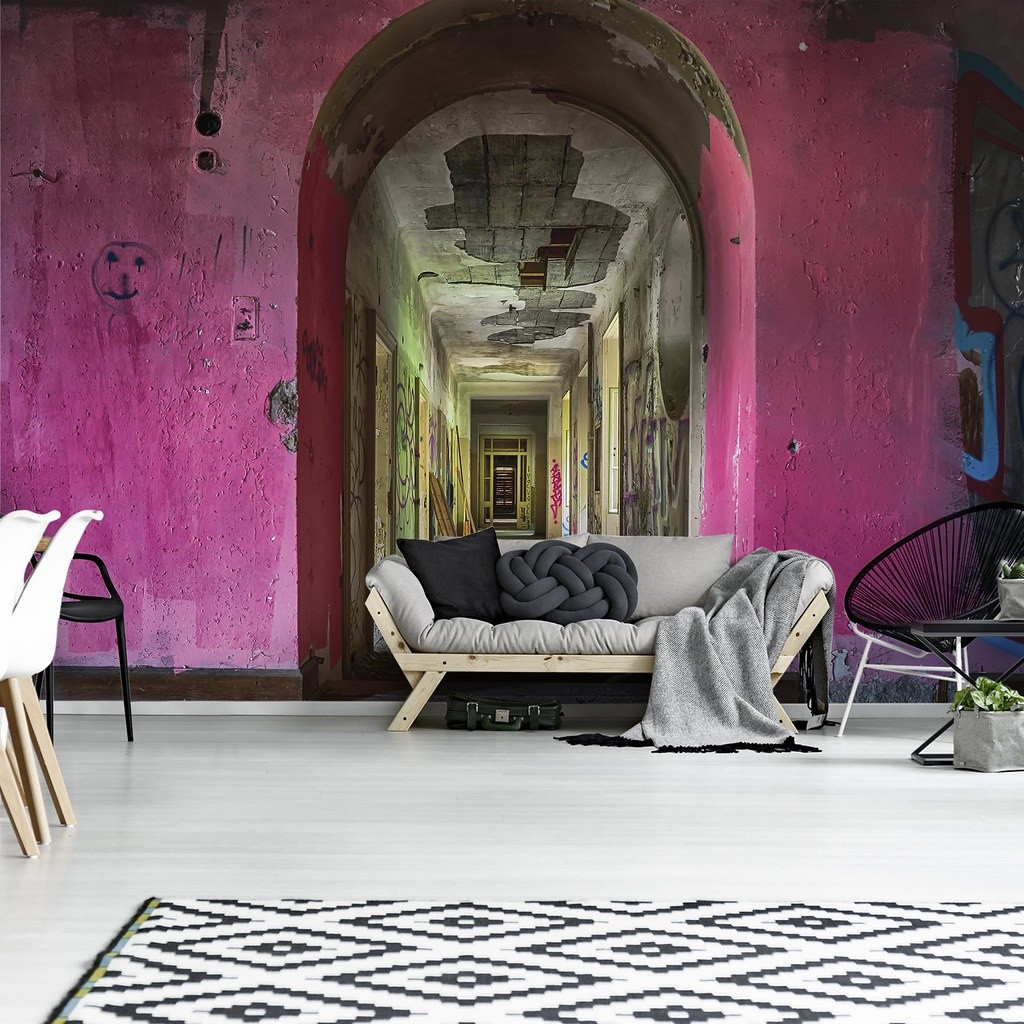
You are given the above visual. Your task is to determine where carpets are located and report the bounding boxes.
[37,892,1024,1024]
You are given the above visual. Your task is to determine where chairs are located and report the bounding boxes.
[27,547,137,747]
[835,500,1024,764]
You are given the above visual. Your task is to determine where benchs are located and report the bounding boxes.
[365,523,835,727]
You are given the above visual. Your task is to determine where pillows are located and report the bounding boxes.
[397,527,509,628]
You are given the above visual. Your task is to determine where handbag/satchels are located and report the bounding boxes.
[444,690,564,731]
[993,561,1024,621]
[953,687,1024,772]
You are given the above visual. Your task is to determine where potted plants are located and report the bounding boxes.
[946,676,1024,774]
